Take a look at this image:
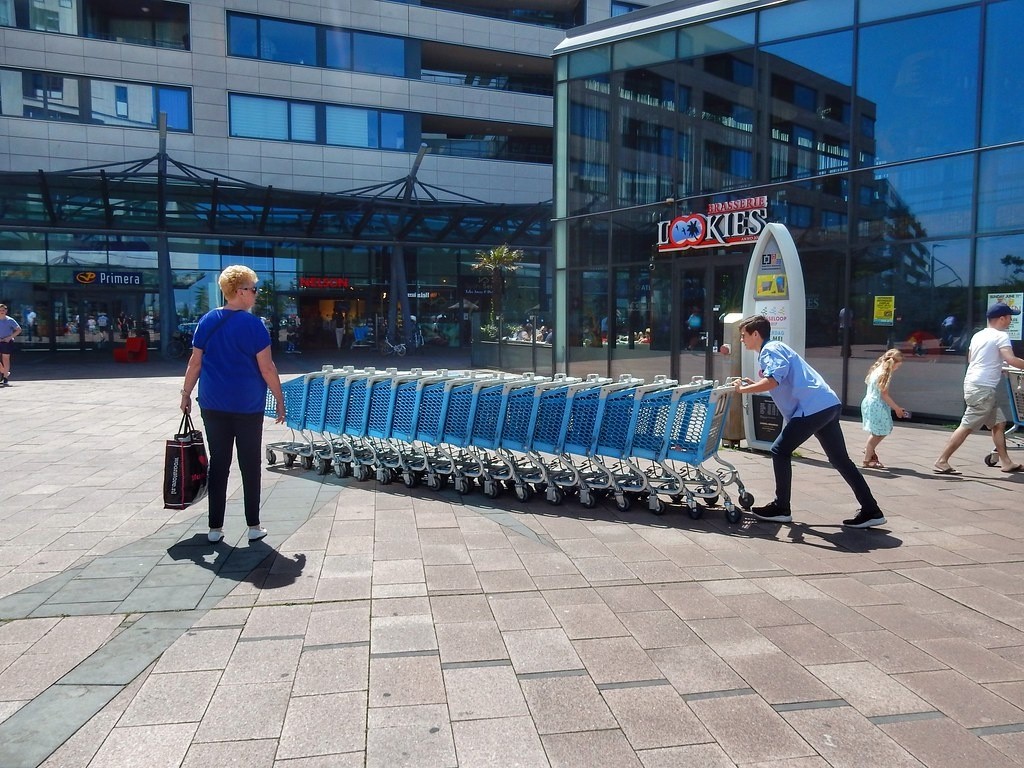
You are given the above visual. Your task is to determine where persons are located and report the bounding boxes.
[862,349,906,469]
[684,306,703,353]
[839,305,855,357]
[255,308,346,354]
[0,304,21,385]
[888,308,954,355]
[504,318,651,348]
[930,303,1024,475]
[728,315,888,527]
[180,265,286,543]
[26,308,159,343]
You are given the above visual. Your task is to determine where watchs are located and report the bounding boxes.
[180,389,191,397]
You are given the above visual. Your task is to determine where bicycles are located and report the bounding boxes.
[381,329,406,357]
[165,333,193,360]
[415,323,424,351]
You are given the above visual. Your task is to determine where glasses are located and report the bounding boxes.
[235,287,257,294]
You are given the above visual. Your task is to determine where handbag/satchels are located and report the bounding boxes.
[163,408,208,510]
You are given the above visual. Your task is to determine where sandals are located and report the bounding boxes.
[863,460,881,469]
[862,448,879,462]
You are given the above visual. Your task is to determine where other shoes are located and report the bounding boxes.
[0,373,4,382]
[3,378,9,384]
[208,531,225,543]
[248,527,267,541]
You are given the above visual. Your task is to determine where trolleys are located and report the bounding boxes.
[263,364,755,525]
[178,323,198,335]
[984,366,1024,466]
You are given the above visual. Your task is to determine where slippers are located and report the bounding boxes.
[933,467,963,475]
[1001,463,1024,472]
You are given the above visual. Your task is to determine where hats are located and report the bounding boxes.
[986,303,1022,317]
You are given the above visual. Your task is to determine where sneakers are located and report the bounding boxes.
[843,507,887,528]
[752,500,792,522]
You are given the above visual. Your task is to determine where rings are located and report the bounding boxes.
[282,416,284,418]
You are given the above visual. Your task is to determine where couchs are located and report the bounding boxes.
[114,335,146,362]
